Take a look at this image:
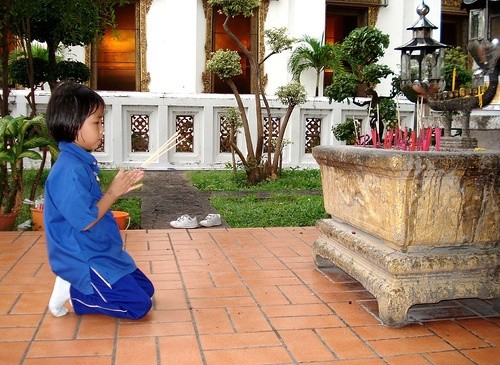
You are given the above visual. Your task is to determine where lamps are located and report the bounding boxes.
[391,0,446,134]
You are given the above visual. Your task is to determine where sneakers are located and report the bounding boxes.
[199,213,222,227]
[170,214,198,229]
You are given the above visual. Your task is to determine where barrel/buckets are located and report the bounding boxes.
[110,211,130,230]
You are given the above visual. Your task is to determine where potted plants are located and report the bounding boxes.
[0,110,54,232]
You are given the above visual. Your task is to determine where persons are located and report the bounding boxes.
[43,82,154,321]
